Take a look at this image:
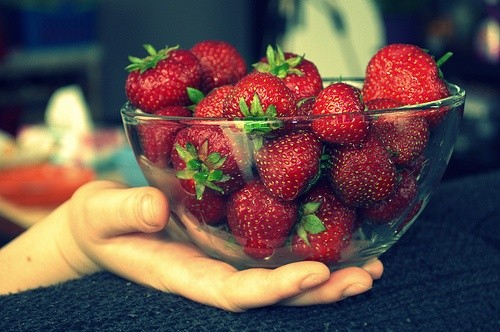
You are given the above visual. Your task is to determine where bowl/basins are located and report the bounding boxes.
[121,77,466,276]
[0,129,124,228]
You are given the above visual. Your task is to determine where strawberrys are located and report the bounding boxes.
[126,41,451,265]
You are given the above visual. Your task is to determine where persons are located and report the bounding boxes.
[91,0,387,129]
[0,178,384,312]
